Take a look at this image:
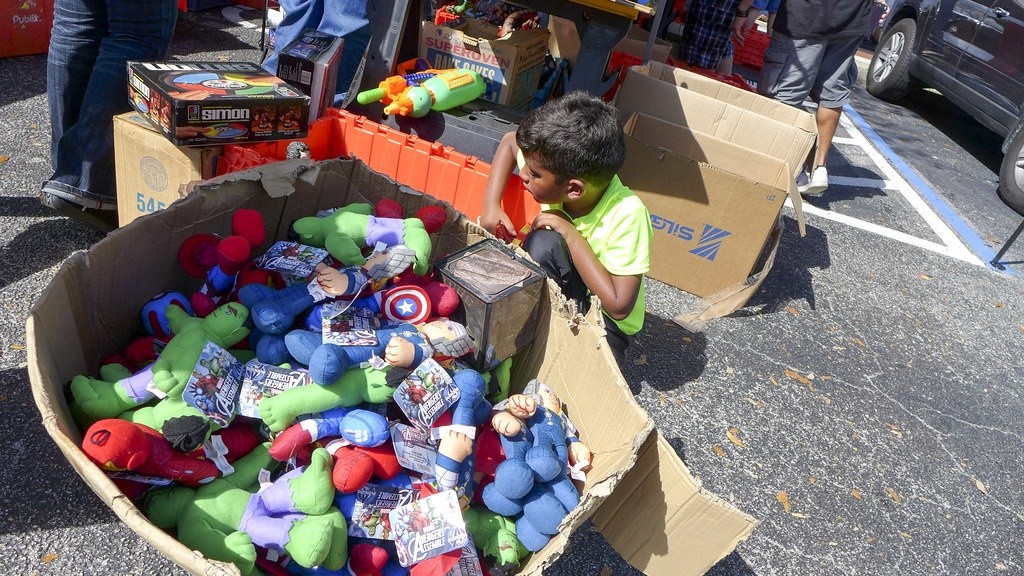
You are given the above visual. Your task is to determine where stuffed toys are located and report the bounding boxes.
[64,197,591,576]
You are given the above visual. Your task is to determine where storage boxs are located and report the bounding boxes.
[601,46,760,110]
[127,62,311,146]
[112,112,219,228]
[418,11,552,111]
[735,27,772,68]
[617,59,819,181]
[622,112,807,303]
[0,0,60,58]
[217,106,542,242]
[431,236,545,373]
[26,153,760,576]
[278,28,344,127]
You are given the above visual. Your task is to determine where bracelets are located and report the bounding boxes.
[735,6,752,18]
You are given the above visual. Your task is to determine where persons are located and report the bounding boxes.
[680,0,875,195]
[481,92,648,369]
[261,0,377,109]
[41,0,179,232]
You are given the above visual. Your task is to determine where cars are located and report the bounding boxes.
[865,0,1024,214]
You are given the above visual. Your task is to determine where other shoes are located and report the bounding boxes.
[41,192,119,235]
[796,166,828,194]
